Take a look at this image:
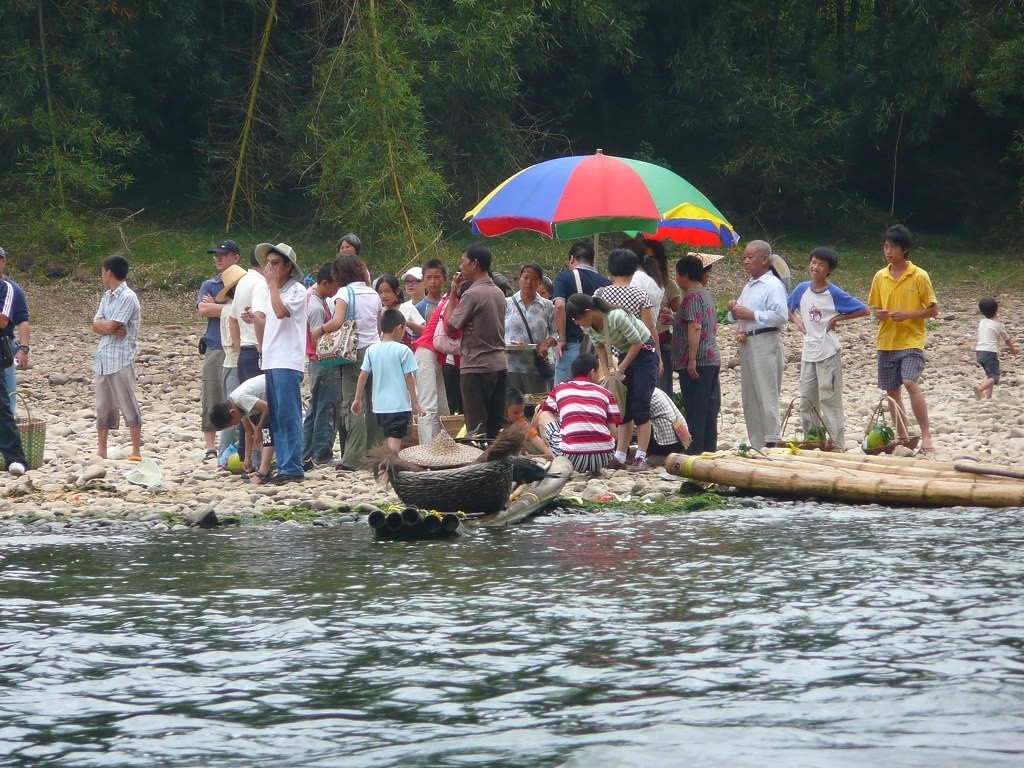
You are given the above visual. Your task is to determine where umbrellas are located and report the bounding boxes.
[463,148,741,269]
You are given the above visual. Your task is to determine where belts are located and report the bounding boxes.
[240,345,257,350]
[740,326,778,337]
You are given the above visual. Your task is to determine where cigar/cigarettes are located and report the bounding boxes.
[456,272,461,273]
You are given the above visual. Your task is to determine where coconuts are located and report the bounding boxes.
[866,428,889,449]
[227,452,244,468]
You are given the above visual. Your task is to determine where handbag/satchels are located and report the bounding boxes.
[600,378,628,418]
[433,320,463,355]
[673,417,694,451]
[315,287,360,369]
[537,357,555,380]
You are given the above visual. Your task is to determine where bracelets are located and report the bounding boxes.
[671,311,673,314]
[20,346,29,352]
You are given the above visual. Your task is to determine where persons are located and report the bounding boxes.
[352,309,426,453]
[196,238,307,484]
[592,249,664,378]
[0,247,30,473]
[726,240,789,449]
[973,298,1018,402]
[647,387,688,456]
[565,293,660,472]
[504,263,559,395]
[786,247,870,453]
[445,245,513,449]
[680,252,725,298]
[643,239,681,398]
[302,234,382,471]
[373,258,463,445]
[552,242,613,386]
[659,255,721,455]
[92,256,143,460]
[534,352,623,475]
[505,387,544,453]
[622,239,664,325]
[867,224,939,451]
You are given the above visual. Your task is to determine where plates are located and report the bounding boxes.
[506,342,538,350]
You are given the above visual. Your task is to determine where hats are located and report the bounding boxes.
[400,267,424,279]
[0,247,5,257]
[207,240,241,256]
[255,242,302,281]
[687,252,725,269]
[214,264,248,302]
[397,428,484,466]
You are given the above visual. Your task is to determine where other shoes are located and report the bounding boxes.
[590,456,647,477]
[201,447,356,485]
[914,445,935,458]
[8,461,25,475]
[975,386,982,401]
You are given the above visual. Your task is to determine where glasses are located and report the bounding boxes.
[404,279,422,285]
[264,258,284,267]
[213,254,235,259]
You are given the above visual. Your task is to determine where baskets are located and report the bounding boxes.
[765,395,842,452]
[862,397,921,458]
[0,392,47,471]
